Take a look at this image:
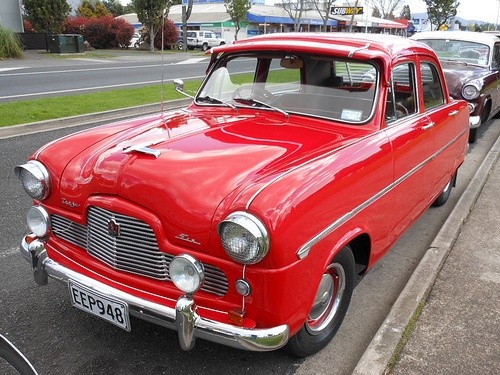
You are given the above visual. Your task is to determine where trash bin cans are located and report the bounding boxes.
[48,32,87,53]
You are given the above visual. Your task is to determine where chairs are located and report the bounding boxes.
[460,50,480,65]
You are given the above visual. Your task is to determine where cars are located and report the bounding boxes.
[14,31,470,358]
[362,31,500,143]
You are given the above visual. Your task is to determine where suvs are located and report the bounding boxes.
[186,31,226,51]
